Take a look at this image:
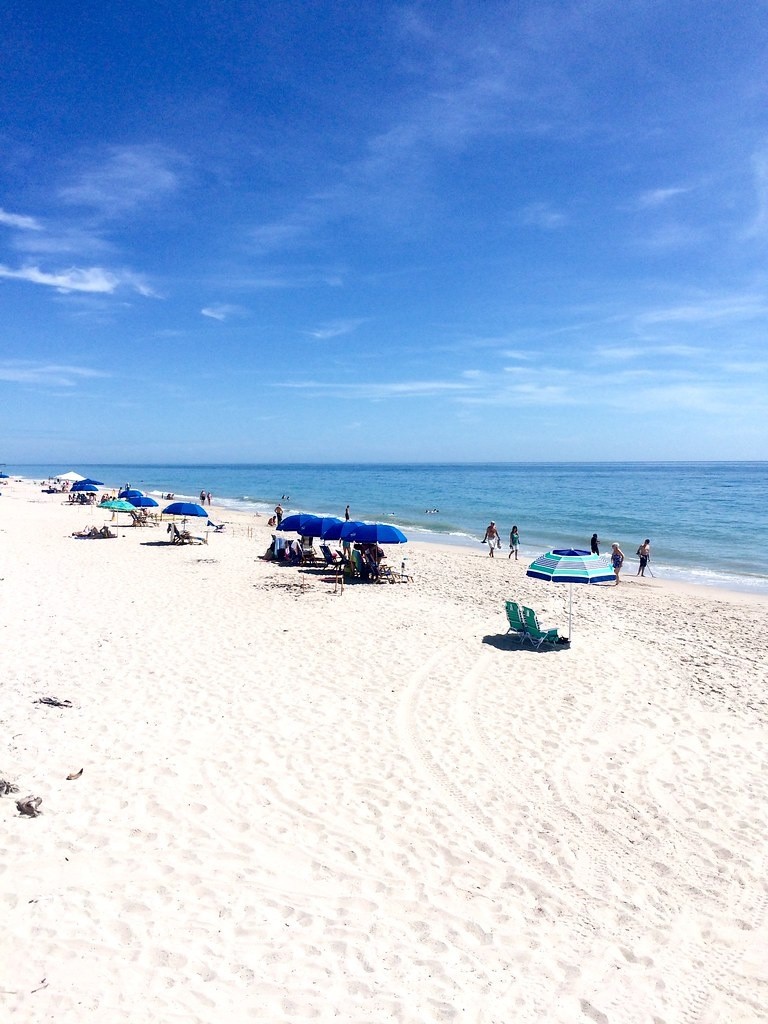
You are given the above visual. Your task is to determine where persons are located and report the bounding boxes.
[636,539,650,577]
[483,521,500,558]
[591,533,600,556]
[100,481,131,500]
[200,489,211,506]
[611,542,624,585]
[41,475,94,504]
[268,494,384,579]
[509,525,520,560]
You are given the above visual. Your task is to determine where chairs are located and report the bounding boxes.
[365,550,412,584]
[336,550,356,573]
[131,510,157,527]
[352,550,400,579]
[300,552,327,567]
[521,606,559,649]
[503,601,525,643]
[319,545,340,572]
[170,523,208,546]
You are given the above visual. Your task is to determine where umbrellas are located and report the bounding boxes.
[161,502,209,532]
[96,489,160,536]
[275,513,407,569]
[526,547,617,641]
[56,471,104,497]
[0,475,9,478]
